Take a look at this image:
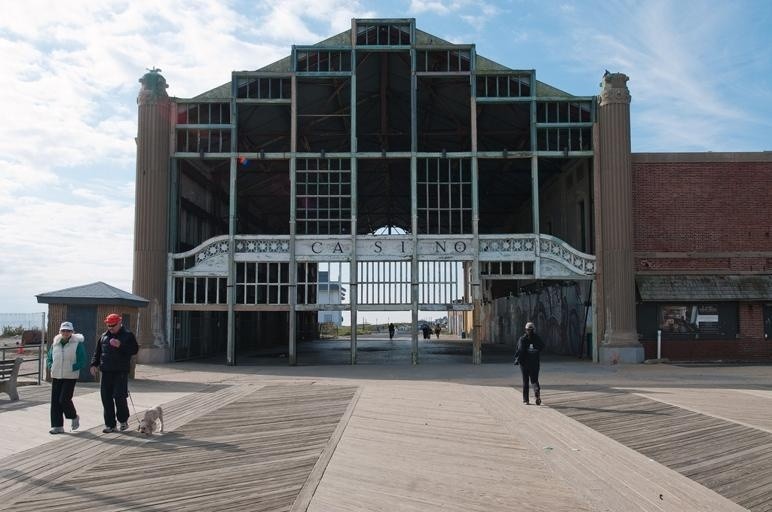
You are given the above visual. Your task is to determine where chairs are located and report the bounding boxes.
[0,357,25,401]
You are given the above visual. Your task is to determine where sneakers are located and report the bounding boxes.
[102,425,118,433]
[119,421,129,432]
[71,415,80,431]
[48,424,65,435]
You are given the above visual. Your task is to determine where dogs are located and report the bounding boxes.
[137,406,164,436]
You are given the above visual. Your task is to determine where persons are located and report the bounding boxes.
[47,322,87,434]
[90,314,138,433]
[389,323,395,338]
[514,322,545,405]
[422,323,441,339]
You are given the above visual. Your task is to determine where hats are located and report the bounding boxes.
[59,321,74,331]
[104,313,122,324]
